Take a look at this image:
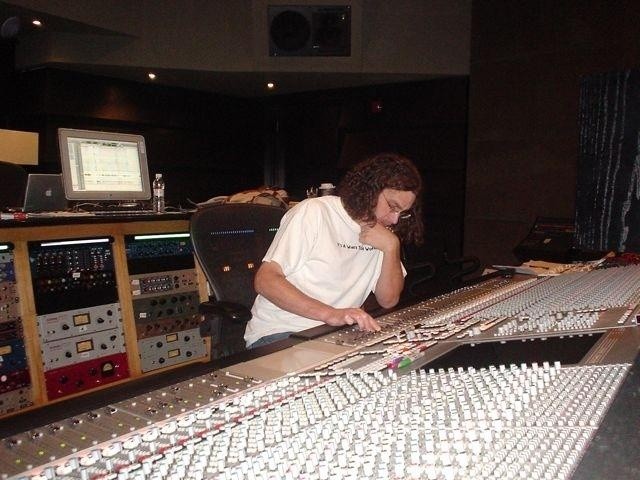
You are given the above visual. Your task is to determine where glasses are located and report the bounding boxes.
[382,190,412,219]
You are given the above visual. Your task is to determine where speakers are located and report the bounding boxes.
[267,5,351,57]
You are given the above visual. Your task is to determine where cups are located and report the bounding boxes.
[318,182,336,195]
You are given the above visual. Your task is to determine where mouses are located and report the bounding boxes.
[166,207,180,213]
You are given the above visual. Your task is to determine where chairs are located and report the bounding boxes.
[182,204,290,359]
[400,218,482,301]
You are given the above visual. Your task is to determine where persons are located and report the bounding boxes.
[239,151,427,353]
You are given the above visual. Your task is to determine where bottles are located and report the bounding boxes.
[152,173,166,214]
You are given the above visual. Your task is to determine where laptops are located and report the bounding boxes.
[9,173,73,212]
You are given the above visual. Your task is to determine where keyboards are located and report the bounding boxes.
[95,210,155,217]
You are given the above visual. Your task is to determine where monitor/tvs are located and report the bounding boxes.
[57,128,151,207]
[411,331,607,372]
